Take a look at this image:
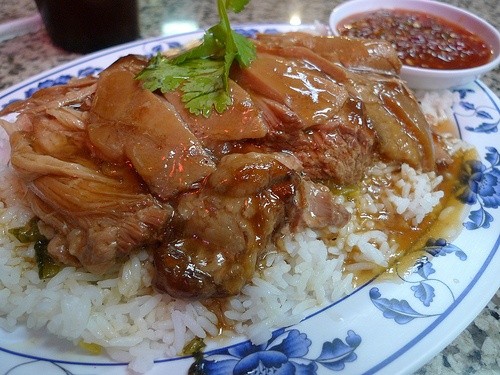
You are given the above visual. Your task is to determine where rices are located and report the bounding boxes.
[1,89,469,374]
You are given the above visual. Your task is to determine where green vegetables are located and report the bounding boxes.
[136,1,257,116]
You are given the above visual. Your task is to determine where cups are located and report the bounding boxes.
[34,1,142,53]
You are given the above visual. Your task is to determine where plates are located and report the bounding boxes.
[0,23,500,375]
[330,0,500,90]
